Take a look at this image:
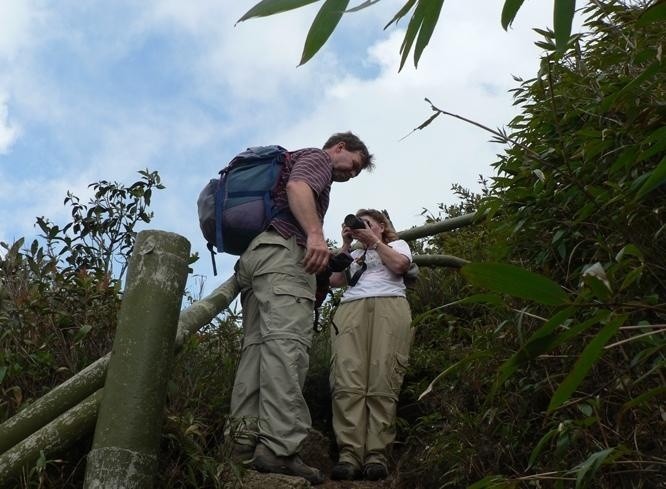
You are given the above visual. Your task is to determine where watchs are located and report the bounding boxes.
[372,240,380,251]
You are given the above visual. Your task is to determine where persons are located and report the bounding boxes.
[327,208,415,482]
[226,131,375,483]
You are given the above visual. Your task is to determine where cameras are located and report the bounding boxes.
[344,213,371,235]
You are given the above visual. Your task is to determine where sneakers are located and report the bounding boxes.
[232,443,323,485]
[331,462,386,480]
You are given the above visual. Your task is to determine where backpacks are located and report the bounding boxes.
[197,145,295,276]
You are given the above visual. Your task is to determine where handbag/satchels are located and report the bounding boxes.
[313,253,354,335]
